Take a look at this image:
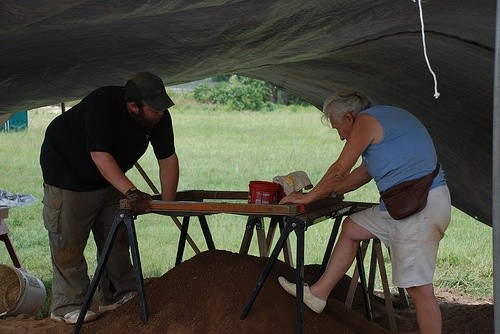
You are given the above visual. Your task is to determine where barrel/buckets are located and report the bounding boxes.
[0,264,46,318]
[248,181,279,204]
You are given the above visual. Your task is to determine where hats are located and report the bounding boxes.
[134,72,175,109]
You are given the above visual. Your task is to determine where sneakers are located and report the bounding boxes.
[278,277,326,313]
[99,291,136,313]
[51,309,96,324]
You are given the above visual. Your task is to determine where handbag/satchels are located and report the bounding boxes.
[381,175,436,221]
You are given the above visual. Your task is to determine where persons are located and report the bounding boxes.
[39,71,179,323]
[279,89,452,333]
